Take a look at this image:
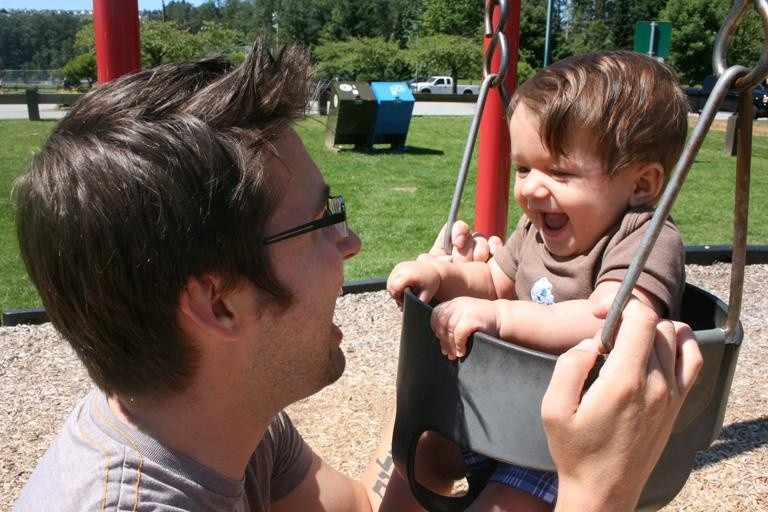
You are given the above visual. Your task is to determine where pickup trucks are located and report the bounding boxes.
[410,75,480,96]
[675,71,768,122]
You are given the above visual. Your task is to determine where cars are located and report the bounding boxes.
[63,76,80,87]
[406,77,427,87]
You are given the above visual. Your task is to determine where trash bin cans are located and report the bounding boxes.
[324,81,414,151]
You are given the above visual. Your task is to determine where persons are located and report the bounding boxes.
[12,35,703,512]
[377,49,689,510]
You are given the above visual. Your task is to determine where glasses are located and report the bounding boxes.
[256,194,352,249]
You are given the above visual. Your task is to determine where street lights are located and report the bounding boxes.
[413,23,420,94]
[0,7,11,18]
[270,11,280,51]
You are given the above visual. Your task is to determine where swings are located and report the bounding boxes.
[391,0,764,511]
